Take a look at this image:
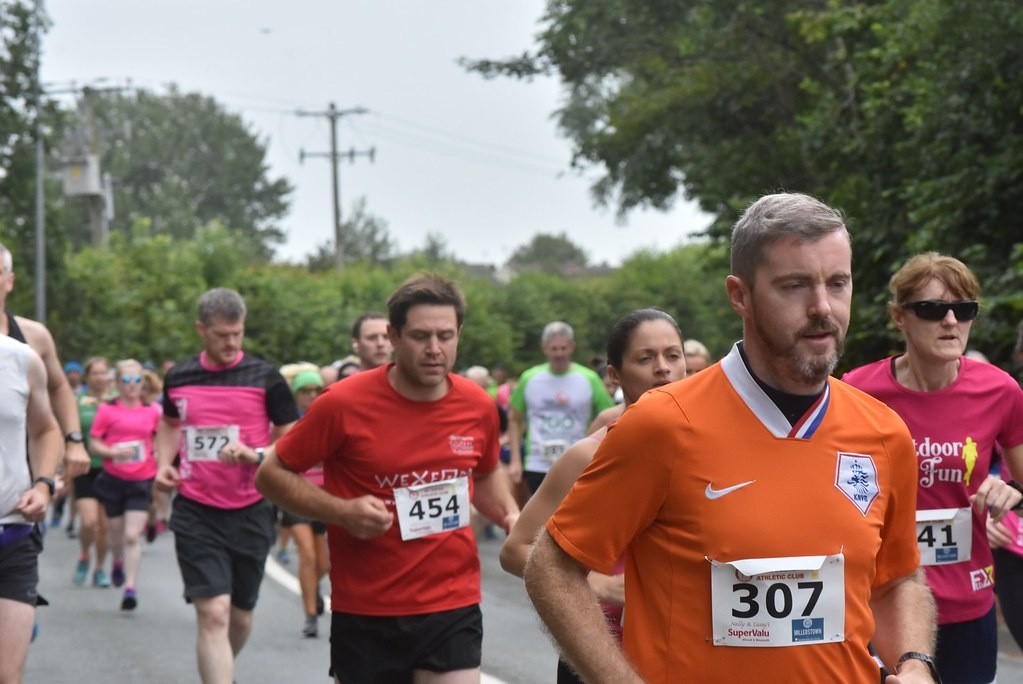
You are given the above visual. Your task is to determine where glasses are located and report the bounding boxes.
[120,375,144,383]
[300,387,324,395]
[898,299,979,321]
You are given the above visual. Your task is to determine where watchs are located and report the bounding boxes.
[36,477,55,496]
[65,432,84,442]
[894,651,942,684]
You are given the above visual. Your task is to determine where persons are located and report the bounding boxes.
[273,312,395,639]
[0,242,189,684]
[844,248,1023,684]
[255,271,521,684]
[525,188,941,684]
[151,286,300,684]
[457,308,712,684]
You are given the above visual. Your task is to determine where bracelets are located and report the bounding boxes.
[255,447,265,463]
[1006,480,1023,510]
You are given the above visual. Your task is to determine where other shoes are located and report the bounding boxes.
[275,550,289,565]
[122,591,135,608]
[147,526,156,542]
[313,592,324,614]
[304,615,319,636]
[73,558,91,582]
[111,559,125,586]
[92,571,110,588]
